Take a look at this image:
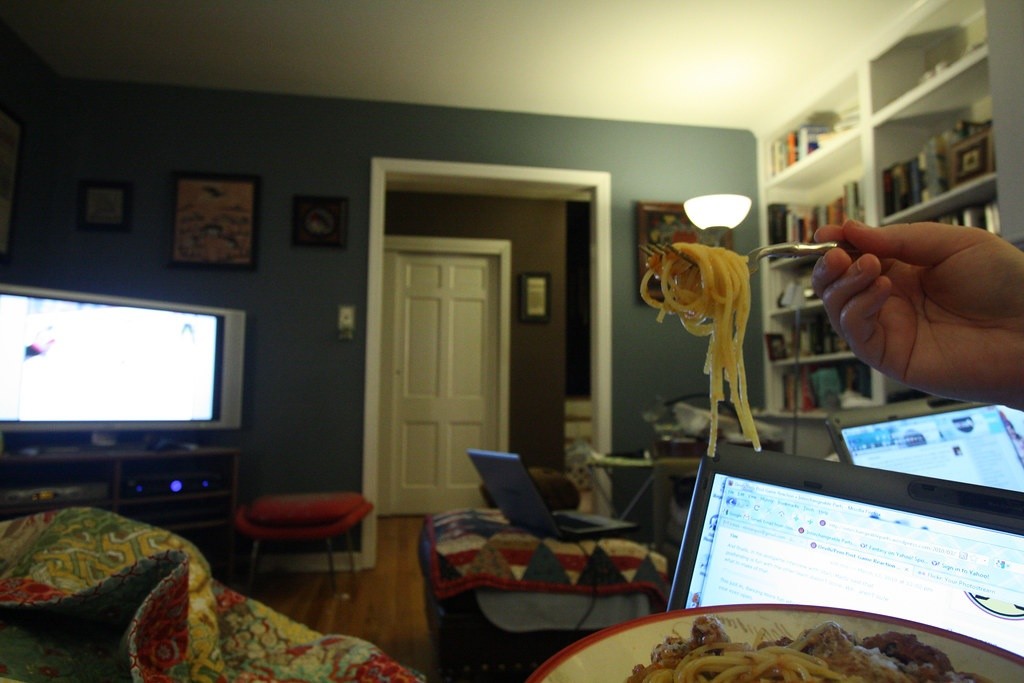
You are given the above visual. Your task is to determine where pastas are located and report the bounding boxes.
[627,627,857,683]
[640,241,760,458]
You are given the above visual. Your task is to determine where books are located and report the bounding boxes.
[764,122,1024,403]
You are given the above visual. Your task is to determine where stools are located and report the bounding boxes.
[232,492,375,599]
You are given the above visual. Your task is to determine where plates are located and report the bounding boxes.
[527,604,1024,683]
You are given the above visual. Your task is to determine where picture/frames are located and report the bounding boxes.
[78,181,133,233]
[633,201,734,304]
[518,270,552,323]
[290,193,347,248]
[164,170,264,272]
[764,332,787,362]
[949,128,992,187]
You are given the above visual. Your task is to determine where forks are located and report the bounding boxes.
[639,242,861,276]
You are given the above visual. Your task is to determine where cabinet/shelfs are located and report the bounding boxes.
[750,0,996,413]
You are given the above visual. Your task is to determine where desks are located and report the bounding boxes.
[588,458,701,520]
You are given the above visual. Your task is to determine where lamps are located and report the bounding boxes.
[686,194,753,245]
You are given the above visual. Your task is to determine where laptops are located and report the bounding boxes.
[465,447,642,541]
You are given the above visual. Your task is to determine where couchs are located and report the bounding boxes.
[417,504,670,683]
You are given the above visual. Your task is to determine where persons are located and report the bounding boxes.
[811,219,1024,412]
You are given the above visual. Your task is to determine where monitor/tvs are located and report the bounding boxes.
[664,442,1024,661]
[0,280,247,437]
[823,393,1024,493]
